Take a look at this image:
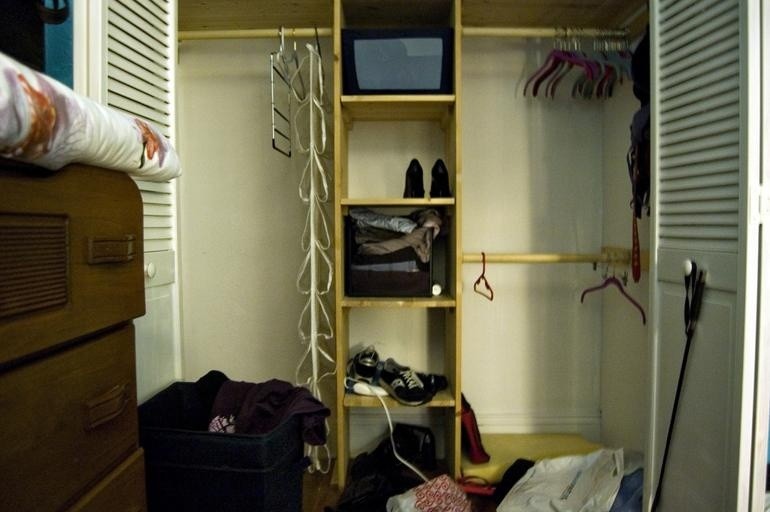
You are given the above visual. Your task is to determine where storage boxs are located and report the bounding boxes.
[138,381,306,512]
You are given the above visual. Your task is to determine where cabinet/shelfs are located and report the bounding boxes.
[0,158,147,511]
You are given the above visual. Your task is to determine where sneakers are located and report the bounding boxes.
[379,358,432,406]
[353,344,379,384]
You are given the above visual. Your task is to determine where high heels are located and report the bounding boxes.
[403,159,424,198]
[430,159,450,196]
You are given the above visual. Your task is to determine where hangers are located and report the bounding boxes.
[177,1,655,492]
[523,22,633,101]
[580,251,646,325]
[473,252,493,300]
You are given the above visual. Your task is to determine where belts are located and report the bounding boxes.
[629,142,640,283]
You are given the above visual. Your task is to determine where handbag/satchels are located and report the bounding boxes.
[461,392,489,463]
[393,423,436,469]
[386,474,472,512]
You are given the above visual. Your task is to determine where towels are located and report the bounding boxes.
[269,22,326,157]
